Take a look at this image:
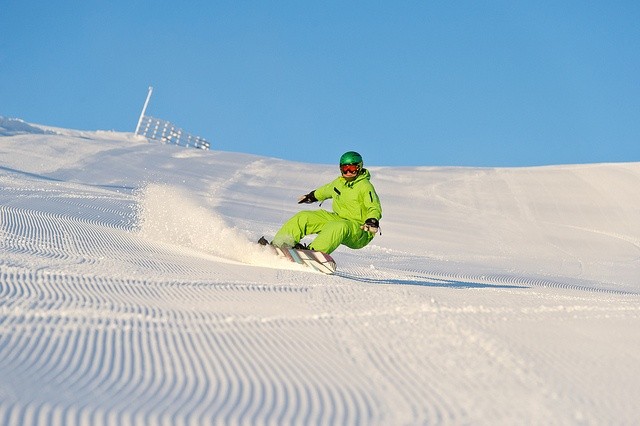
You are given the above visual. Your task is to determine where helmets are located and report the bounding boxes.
[339,152,363,176]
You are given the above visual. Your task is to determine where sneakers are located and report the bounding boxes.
[294,243,310,250]
[258,236,269,245]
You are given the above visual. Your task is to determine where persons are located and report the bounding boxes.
[258,152,382,255]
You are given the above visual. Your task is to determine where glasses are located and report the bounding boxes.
[340,160,362,174]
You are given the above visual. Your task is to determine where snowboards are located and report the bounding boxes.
[271,247,337,275]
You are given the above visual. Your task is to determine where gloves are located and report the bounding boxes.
[297,190,318,204]
[359,218,381,235]
